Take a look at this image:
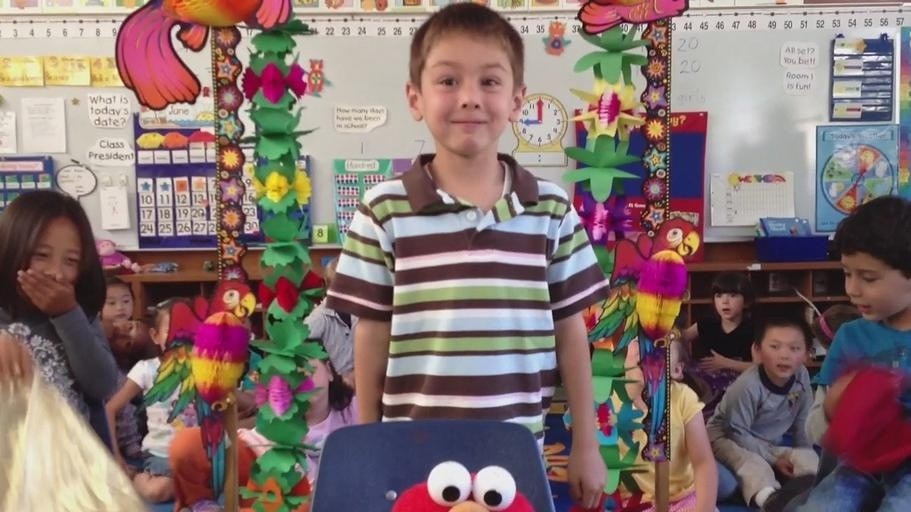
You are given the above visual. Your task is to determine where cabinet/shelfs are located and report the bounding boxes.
[679,259,851,370]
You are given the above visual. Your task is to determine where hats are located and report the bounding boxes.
[829,368,911,473]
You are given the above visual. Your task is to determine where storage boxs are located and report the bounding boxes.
[753,235,831,263]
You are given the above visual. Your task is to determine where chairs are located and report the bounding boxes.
[310,417,555,512]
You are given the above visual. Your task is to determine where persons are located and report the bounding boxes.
[326,3,606,510]
[612,273,815,512]
[797,196,910,511]
[97,259,359,512]
[1,188,118,456]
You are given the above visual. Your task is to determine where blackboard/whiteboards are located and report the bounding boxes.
[0,2,911,252]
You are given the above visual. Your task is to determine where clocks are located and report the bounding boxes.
[509,92,569,168]
